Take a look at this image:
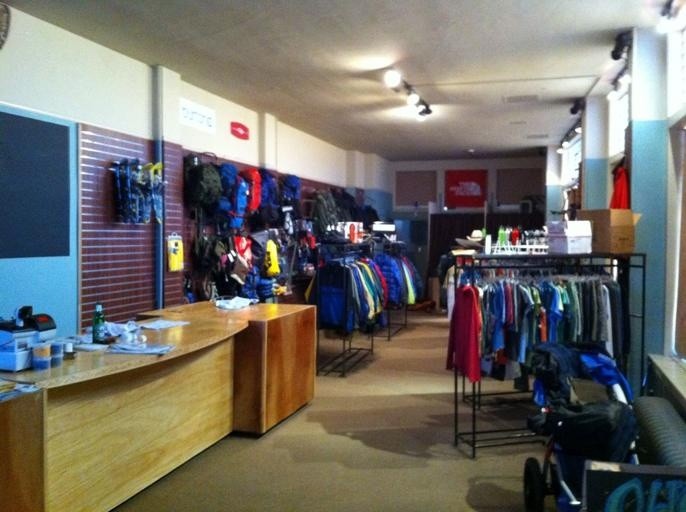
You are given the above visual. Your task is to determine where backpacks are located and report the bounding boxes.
[190,163,299,229]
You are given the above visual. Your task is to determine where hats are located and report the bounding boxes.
[466,229,484,241]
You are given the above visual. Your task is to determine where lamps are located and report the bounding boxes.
[570,99,584,114]
[610,31,631,61]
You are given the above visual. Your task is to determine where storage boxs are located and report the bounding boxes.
[546,209,642,255]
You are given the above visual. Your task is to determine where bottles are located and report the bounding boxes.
[92,302,106,343]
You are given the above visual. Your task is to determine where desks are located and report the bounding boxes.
[0,315,253,512]
